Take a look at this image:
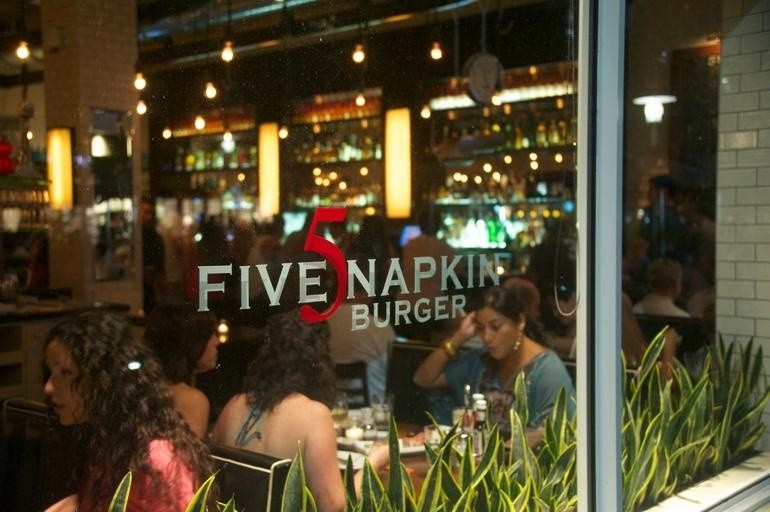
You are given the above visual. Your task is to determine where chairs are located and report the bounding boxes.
[334,361,370,407]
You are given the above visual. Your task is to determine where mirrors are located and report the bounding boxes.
[88,109,134,284]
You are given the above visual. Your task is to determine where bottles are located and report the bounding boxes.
[470,393,493,461]
[172,113,578,251]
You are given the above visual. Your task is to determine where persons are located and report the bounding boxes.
[133,299,221,439]
[206,305,402,512]
[98,180,718,397]
[37,308,226,512]
[412,276,576,458]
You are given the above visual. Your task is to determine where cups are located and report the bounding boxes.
[372,392,396,430]
[328,392,349,437]
[451,407,469,438]
[424,422,454,445]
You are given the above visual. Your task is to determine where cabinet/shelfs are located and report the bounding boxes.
[149,89,578,248]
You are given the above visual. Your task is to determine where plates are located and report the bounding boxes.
[397,436,438,456]
[336,449,367,471]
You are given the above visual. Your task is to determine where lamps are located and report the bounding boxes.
[45,124,75,212]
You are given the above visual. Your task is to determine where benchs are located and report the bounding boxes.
[633,312,714,354]
[1,395,291,512]
[383,340,645,446]
